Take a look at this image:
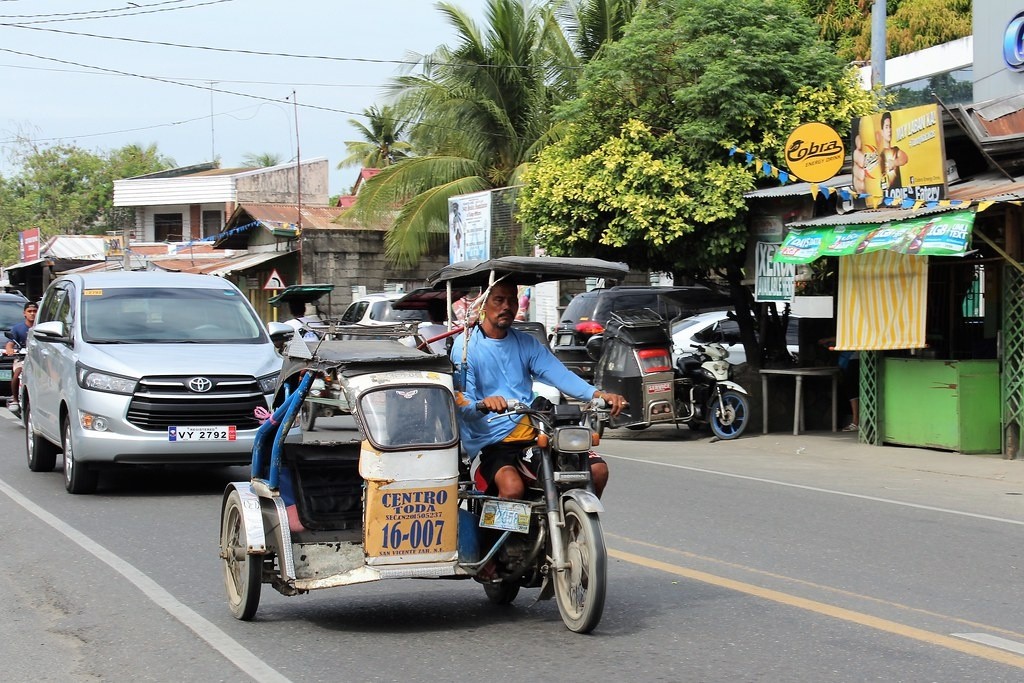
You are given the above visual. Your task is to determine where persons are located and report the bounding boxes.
[853,112,910,209]
[6,302,39,412]
[284,299,329,341]
[449,280,627,581]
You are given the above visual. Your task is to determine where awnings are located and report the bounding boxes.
[4,259,44,272]
[153,244,299,278]
[745,174,851,196]
[784,173,1024,226]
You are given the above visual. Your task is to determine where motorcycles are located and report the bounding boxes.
[217,257,633,634]
[672,321,750,440]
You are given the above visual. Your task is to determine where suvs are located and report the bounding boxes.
[0,289,33,396]
[550,286,733,431]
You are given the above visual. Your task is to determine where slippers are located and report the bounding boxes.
[477,560,499,586]
[8,402,20,412]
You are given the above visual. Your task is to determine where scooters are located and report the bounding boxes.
[3,332,27,421]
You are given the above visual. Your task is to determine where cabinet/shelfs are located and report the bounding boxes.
[876,354,1003,454]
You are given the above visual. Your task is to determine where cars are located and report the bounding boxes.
[21,270,285,494]
[670,310,800,370]
[337,291,433,341]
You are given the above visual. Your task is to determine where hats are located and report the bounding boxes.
[23,302,39,310]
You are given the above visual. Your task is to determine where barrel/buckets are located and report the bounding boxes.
[756,215,784,243]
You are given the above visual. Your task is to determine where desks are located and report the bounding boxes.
[759,368,840,436]
